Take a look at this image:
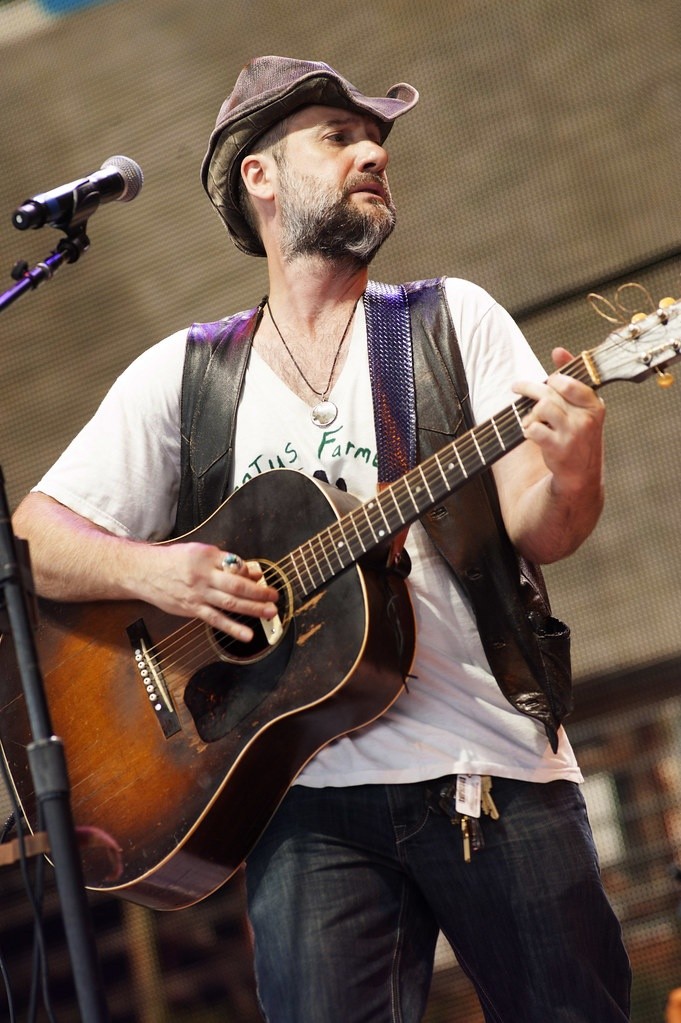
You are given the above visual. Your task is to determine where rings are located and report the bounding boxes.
[222,556,241,573]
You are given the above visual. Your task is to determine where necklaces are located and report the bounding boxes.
[266,293,361,426]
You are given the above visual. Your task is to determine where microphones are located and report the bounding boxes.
[12,156,144,229]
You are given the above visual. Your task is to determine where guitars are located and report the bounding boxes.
[0,295,681,914]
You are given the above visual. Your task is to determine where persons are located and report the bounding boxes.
[10,58,632,1023]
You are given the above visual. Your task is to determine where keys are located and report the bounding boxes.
[440,776,498,862]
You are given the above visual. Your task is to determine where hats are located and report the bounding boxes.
[200,56,419,258]
